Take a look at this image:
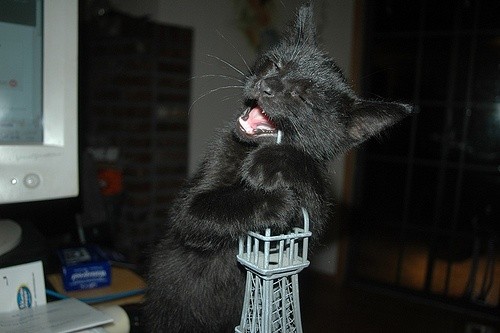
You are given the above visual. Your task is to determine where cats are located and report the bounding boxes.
[134,0,416,333]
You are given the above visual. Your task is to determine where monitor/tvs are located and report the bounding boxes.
[0,0,79,204]
